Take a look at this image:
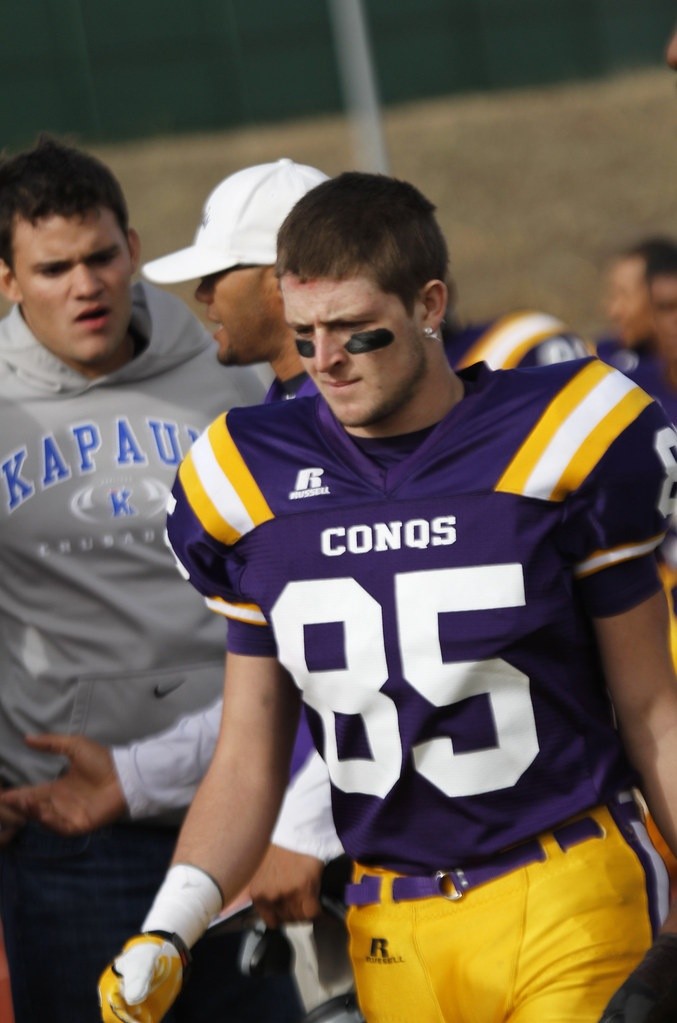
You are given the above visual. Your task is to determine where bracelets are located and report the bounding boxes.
[141,864,225,951]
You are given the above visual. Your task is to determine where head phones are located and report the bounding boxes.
[240,895,367,1023]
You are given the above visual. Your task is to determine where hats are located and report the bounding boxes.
[140,158,334,285]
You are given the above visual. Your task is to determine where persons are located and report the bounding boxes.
[0,133,677,1023]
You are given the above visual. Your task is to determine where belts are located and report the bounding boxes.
[345,799,640,906]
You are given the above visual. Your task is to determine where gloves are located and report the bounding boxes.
[97,931,192,1023]
